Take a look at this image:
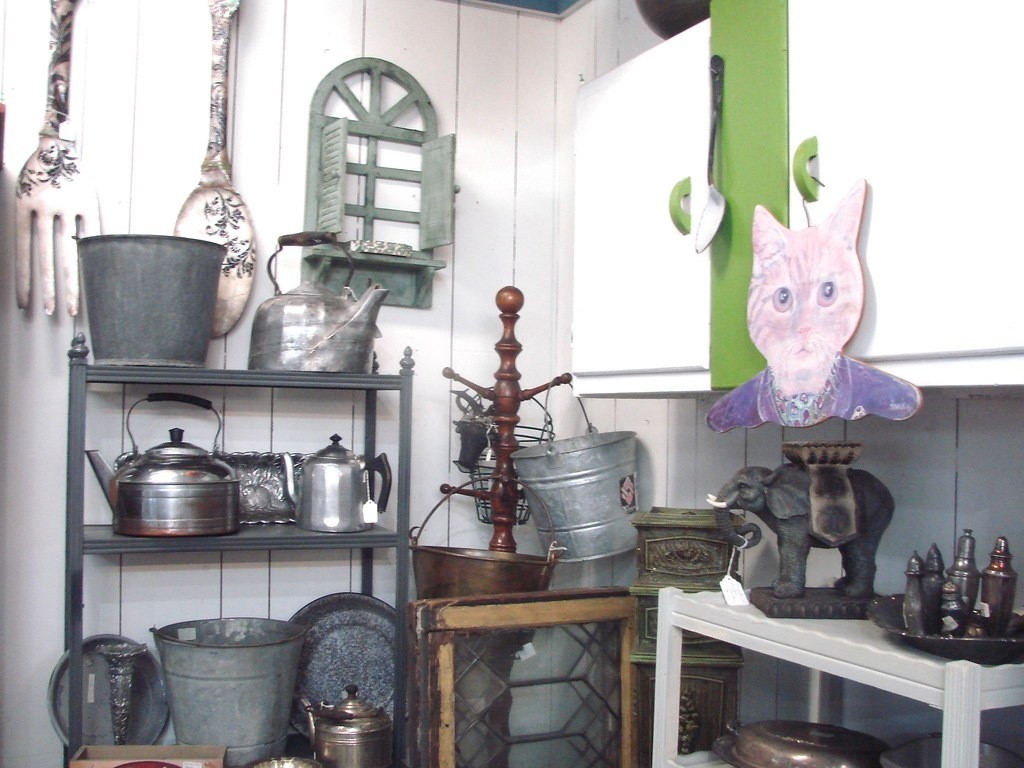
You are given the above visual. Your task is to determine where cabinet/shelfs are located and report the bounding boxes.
[50,332,413,768]
[650,582,1024,767]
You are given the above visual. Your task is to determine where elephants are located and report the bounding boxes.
[706,463,896,600]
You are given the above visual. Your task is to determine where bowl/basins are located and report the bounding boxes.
[635,0,710,40]
[243,755,323,768]
[879,735,1024,768]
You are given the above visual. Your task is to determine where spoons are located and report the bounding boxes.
[694,55,727,254]
[174,0,254,336]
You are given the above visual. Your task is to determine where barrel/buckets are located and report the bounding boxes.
[151,615,306,768]
[77,233,228,369]
[510,377,637,562]
[410,474,563,646]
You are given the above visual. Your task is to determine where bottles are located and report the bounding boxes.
[941,528,981,617]
[939,580,961,638]
[903,548,927,635]
[981,536,1017,637]
[920,541,946,635]
[959,609,985,638]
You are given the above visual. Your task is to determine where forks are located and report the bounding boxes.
[16,0,102,320]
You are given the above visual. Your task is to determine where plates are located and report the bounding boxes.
[48,632,168,752]
[865,592,1023,667]
[288,592,399,734]
[712,732,743,768]
[114,446,306,526]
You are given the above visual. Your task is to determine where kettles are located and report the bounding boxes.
[85,392,240,536]
[296,683,393,768]
[245,231,390,375]
[281,434,391,534]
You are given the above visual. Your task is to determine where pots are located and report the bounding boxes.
[724,719,884,768]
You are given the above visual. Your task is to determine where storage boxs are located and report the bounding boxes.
[245,229,387,378]
[905,524,1019,637]
[409,475,567,661]
[72,231,230,367]
